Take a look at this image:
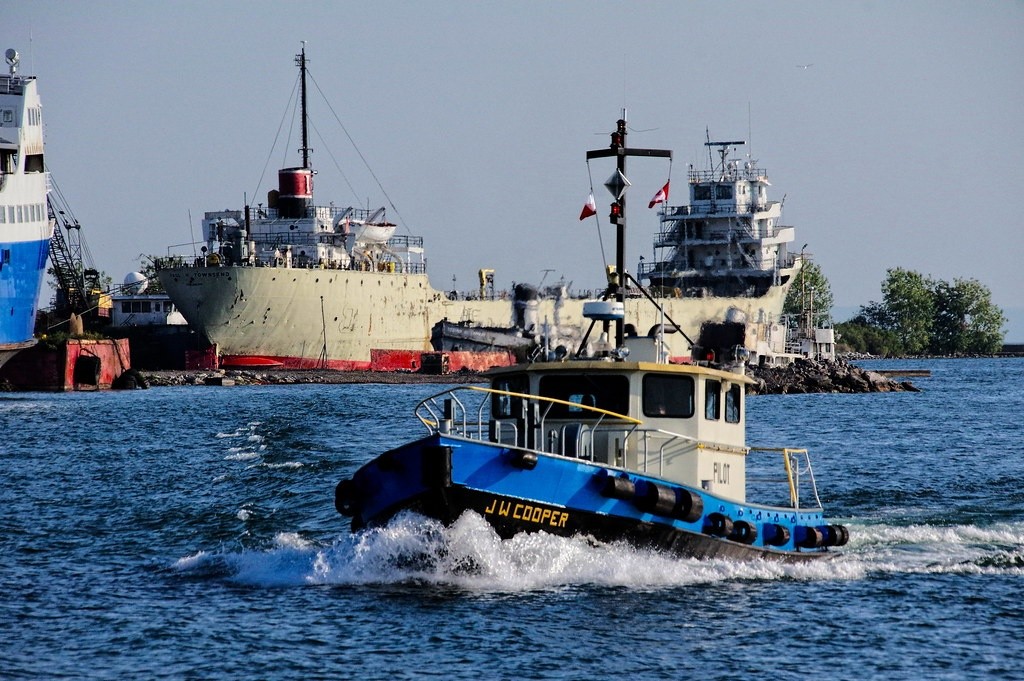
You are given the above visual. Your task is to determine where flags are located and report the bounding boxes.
[579,192,596,220]
[648,183,668,208]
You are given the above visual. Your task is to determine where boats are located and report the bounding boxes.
[0,49,57,371]
[779,287,836,365]
[429,317,535,359]
[68,286,114,324]
[111,295,220,370]
[148,39,805,373]
[334,108,850,565]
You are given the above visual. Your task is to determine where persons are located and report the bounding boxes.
[705,252,713,268]
[296,250,305,267]
[548,345,565,362]
